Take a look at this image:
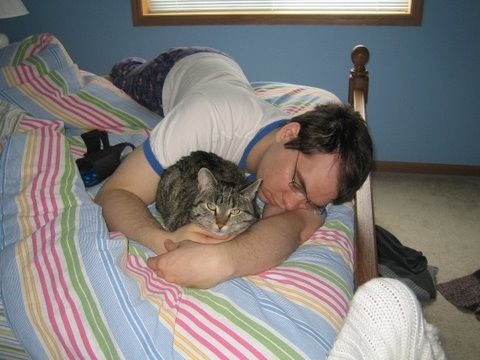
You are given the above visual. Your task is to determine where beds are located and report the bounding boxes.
[0,33,375,360]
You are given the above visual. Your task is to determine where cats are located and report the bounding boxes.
[155,150,262,235]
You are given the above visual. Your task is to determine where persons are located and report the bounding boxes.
[94,46,373,289]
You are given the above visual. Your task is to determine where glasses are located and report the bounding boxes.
[288,150,327,215]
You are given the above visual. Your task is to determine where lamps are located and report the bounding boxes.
[0,0,29,19]
